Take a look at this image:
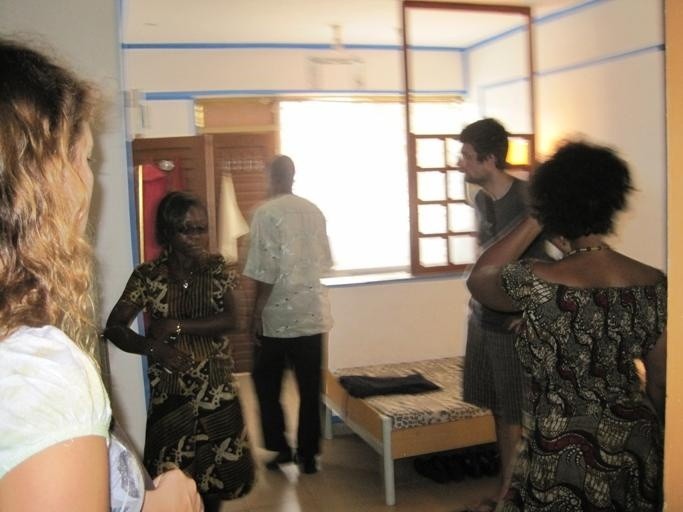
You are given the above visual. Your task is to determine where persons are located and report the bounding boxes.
[0,39,205,512]
[104,191,256,512]
[456,118,572,512]
[241,155,334,474]
[466,140,667,512]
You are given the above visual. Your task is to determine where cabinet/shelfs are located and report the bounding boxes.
[130,131,279,377]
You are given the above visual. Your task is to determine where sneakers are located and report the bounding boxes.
[266,448,321,474]
[414,446,501,483]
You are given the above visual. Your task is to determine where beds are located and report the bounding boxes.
[327,353,496,506]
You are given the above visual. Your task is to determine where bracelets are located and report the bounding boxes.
[176,320,184,336]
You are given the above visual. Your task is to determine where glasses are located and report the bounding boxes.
[175,221,209,235]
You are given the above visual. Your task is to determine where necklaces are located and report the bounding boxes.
[564,245,610,258]
